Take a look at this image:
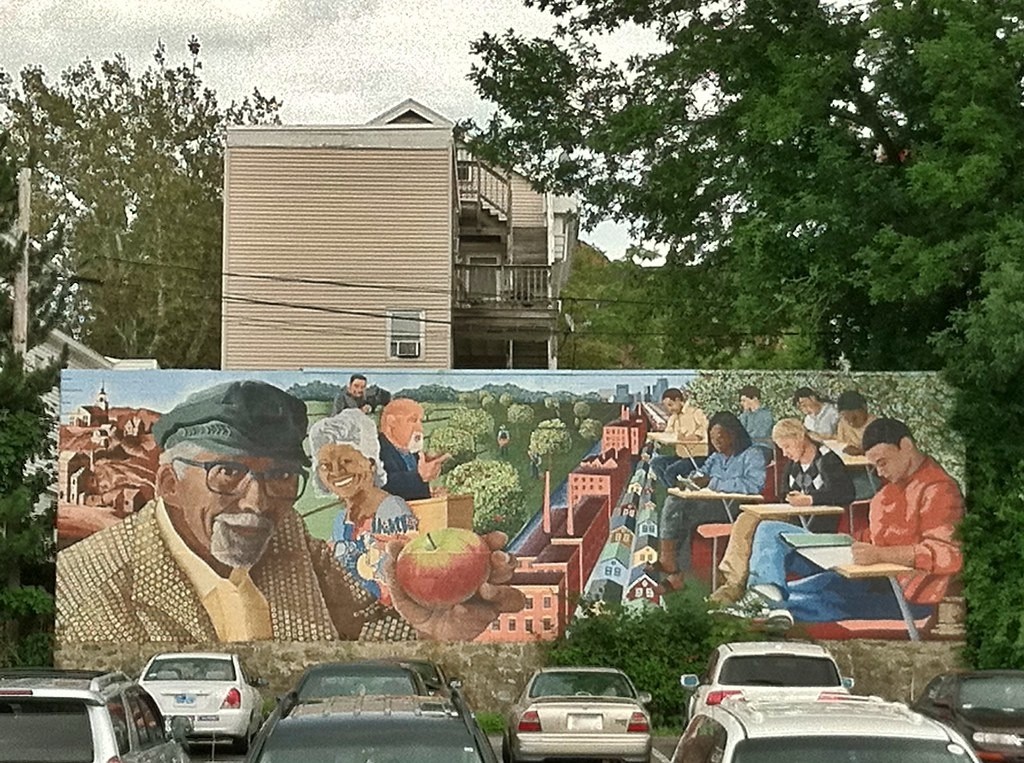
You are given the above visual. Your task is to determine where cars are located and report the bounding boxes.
[501,667,654,763]
[138,652,267,754]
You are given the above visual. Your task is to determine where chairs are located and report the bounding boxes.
[647,432,951,642]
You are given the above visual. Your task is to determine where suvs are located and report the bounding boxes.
[0,669,193,763]
[245,662,499,762]
[667,642,1024,762]
